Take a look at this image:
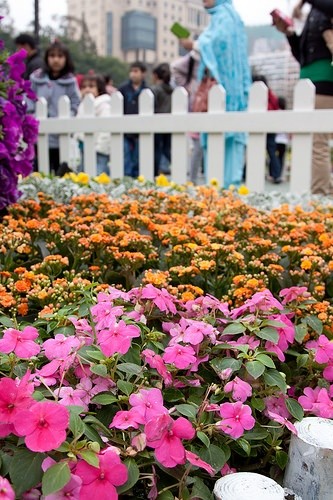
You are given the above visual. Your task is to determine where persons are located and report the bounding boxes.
[178,0,251,191]
[299,4,333,198]
[271,0,333,64]
[4,33,289,184]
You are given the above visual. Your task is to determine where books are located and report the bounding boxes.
[270,9,294,28]
[171,22,191,41]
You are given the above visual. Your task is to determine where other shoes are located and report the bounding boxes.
[272,178,281,183]
